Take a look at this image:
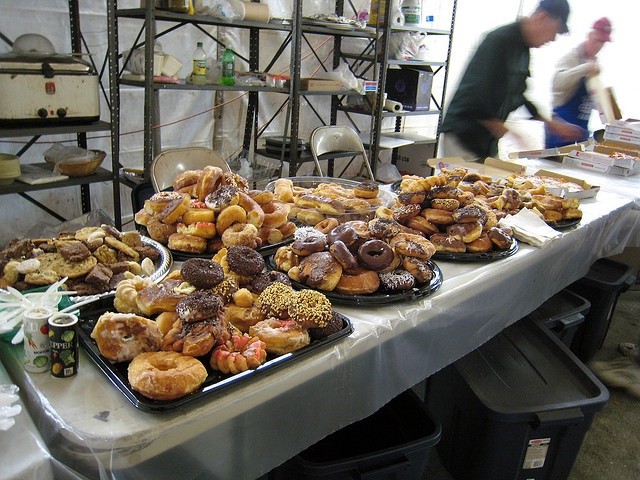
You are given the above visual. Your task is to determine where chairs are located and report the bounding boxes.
[150,146,232,194]
[308,126,375,181]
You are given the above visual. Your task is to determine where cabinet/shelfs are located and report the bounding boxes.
[116,0,297,190]
[254,0,380,192]
[0,0,120,231]
[330,0,458,187]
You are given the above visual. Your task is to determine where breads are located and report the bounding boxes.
[1,224,160,298]
[375,167,583,252]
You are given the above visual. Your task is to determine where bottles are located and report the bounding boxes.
[221,45,235,83]
[193,41,206,85]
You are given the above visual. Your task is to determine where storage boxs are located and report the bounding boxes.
[424,312,610,480]
[531,288,592,349]
[387,65,435,112]
[288,387,442,480]
[567,257,638,364]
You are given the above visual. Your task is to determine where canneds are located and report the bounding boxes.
[48,313,80,379]
[23,307,53,373]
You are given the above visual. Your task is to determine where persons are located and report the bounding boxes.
[439,0,586,161]
[546,16,611,149]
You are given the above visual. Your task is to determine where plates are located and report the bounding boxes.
[140,211,295,264]
[430,236,519,262]
[264,175,397,214]
[61,236,173,303]
[544,208,583,229]
[271,259,444,305]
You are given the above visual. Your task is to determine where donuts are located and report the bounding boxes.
[90,246,343,401]
[272,178,382,225]
[275,218,436,295]
[135,165,298,254]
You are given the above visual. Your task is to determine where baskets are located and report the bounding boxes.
[44,149,106,177]
[217,147,279,182]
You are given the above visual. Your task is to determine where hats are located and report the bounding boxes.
[592,17,612,42]
[541,0,571,34]
